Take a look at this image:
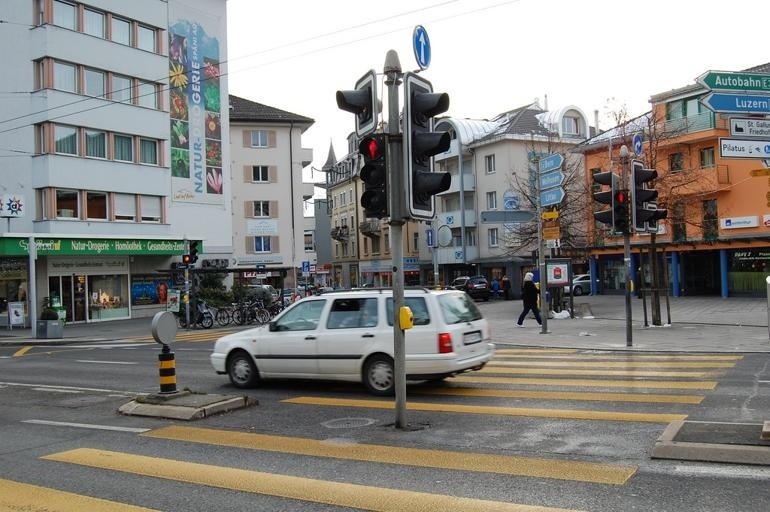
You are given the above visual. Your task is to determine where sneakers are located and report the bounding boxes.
[536,324,543,328]
[516,323,526,328]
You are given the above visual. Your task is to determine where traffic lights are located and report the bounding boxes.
[404,71,452,220]
[360,136,390,219]
[632,160,668,233]
[592,172,613,225]
[616,190,627,230]
[183,255,192,264]
[336,72,377,135]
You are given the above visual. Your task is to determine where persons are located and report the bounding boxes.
[516,272,544,328]
[491,275,513,300]
[155,281,171,305]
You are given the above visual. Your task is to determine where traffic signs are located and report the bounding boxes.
[538,151,567,208]
[693,69,770,172]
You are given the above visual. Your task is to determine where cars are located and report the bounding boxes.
[211,285,494,395]
[562,273,591,297]
[447,274,491,303]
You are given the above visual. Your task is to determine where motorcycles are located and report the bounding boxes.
[178,299,213,329]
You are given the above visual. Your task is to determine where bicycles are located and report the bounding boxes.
[200,299,285,326]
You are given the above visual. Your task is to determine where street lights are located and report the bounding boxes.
[619,144,634,190]
